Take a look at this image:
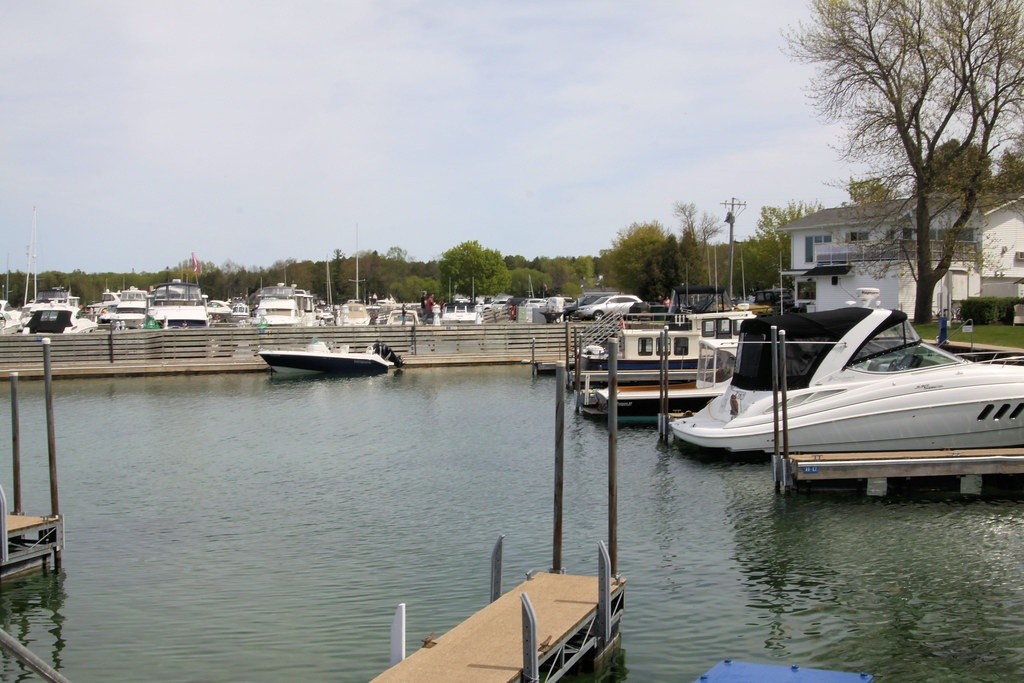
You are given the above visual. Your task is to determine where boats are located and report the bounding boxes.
[257,341,394,381]
[668,306,1024,452]
[579,303,757,385]
[578,339,738,424]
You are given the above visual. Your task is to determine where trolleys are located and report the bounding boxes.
[539,311,563,324]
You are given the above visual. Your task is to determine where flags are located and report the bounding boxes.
[192,253,201,272]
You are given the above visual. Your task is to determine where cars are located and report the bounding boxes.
[491,300,507,311]
[578,295,643,321]
[545,296,576,321]
[475,296,497,312]
[502,298,525,319]
[454,296,472,303]
[521,299,546,308]
[712,299,774,317]
[562,296,604,321]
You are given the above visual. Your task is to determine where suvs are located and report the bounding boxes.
[754,288,794,311]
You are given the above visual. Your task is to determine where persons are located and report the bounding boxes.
[420,291,436,325]
[663,296,671,313]
[508,304,517,322]
[402,304,407,324]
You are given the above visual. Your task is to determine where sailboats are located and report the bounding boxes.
[0,206,484,334]
